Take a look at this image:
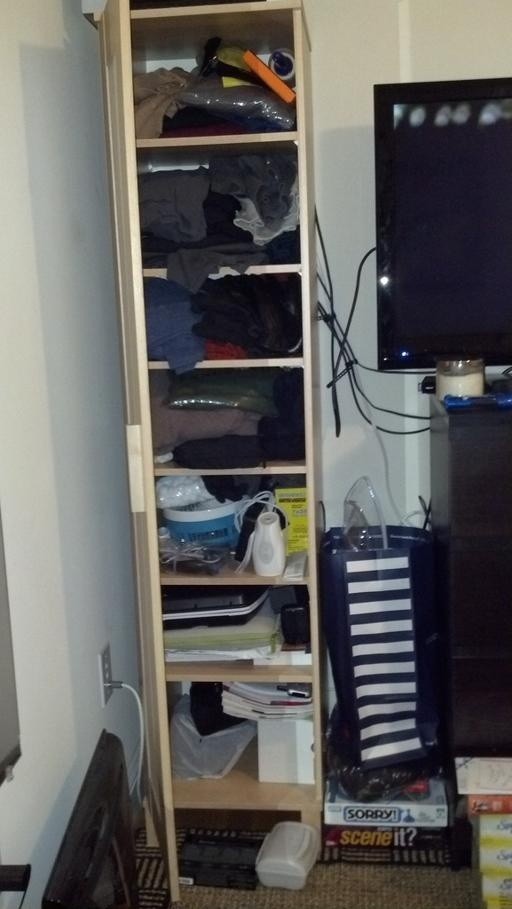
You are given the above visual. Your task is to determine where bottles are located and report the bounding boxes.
[433,354,487,403]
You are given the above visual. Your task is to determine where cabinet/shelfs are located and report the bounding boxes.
[424,374,512,760]
[98,0,333,909]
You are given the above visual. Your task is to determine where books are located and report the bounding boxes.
[222,678,314,720]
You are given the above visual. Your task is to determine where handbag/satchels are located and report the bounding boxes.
[319,497,441,771]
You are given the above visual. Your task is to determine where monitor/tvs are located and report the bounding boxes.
[373,77,512,370]
